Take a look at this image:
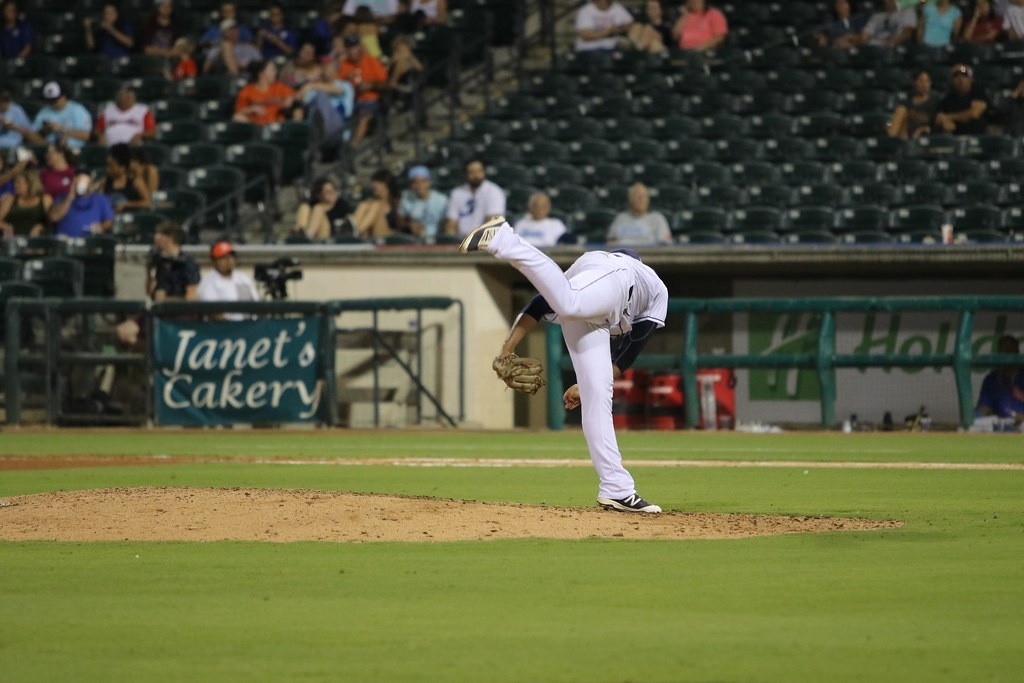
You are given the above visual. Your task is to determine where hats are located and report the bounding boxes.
[43,80,65,106]
[409,165,432,181]
[209,241,237,259]
[613,247,641,261]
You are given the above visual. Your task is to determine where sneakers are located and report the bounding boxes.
[459,215,506,253]
[597,493,662,514]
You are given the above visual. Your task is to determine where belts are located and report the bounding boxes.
[623,286,633,315]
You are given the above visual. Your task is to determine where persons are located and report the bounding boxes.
[811,0,1024,58]
[884,62,1024,142]
[573,0,729,62]
[292,156,675,249]
[456,214,669,514]
[0,80,157,240]
[145,220,261,324]
[0,0,448,142]
[957,334,1024,434]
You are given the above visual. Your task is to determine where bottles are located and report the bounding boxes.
[920,413,932,432]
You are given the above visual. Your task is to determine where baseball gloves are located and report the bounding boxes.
[491,353,546,396]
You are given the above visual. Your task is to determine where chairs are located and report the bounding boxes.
[0,3,1023,294]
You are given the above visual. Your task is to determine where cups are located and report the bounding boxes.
[76,174,92,196]
[940,223,954,245]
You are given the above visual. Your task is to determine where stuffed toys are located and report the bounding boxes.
[85,312,147,403]
